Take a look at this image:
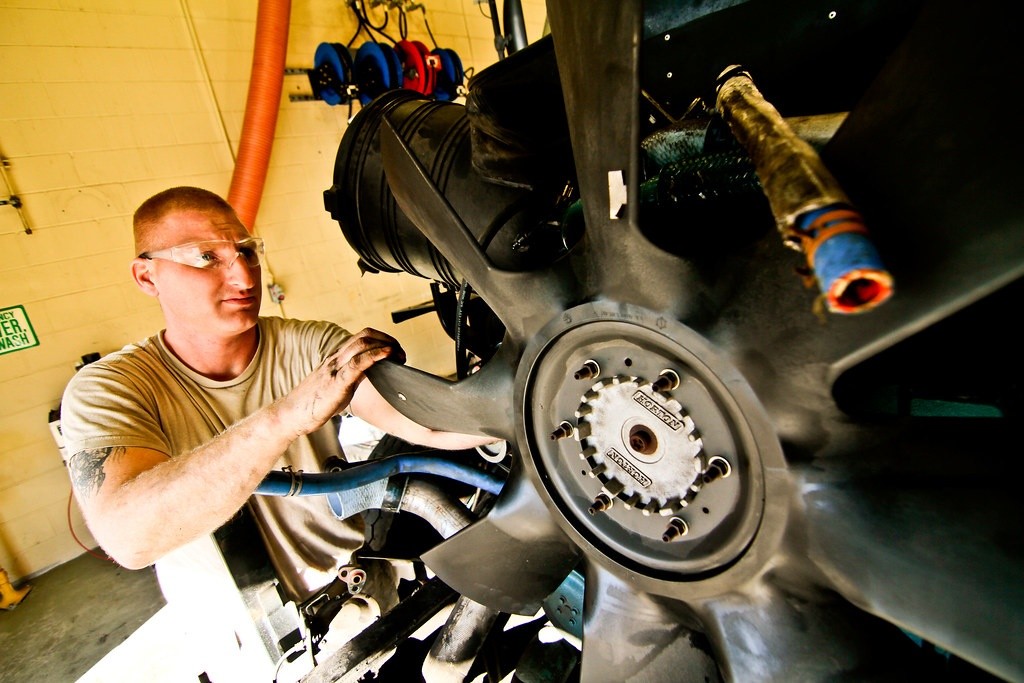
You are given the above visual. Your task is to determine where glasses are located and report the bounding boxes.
[138,237,264,271]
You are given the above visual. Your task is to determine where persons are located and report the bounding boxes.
[61,187,505,601]
